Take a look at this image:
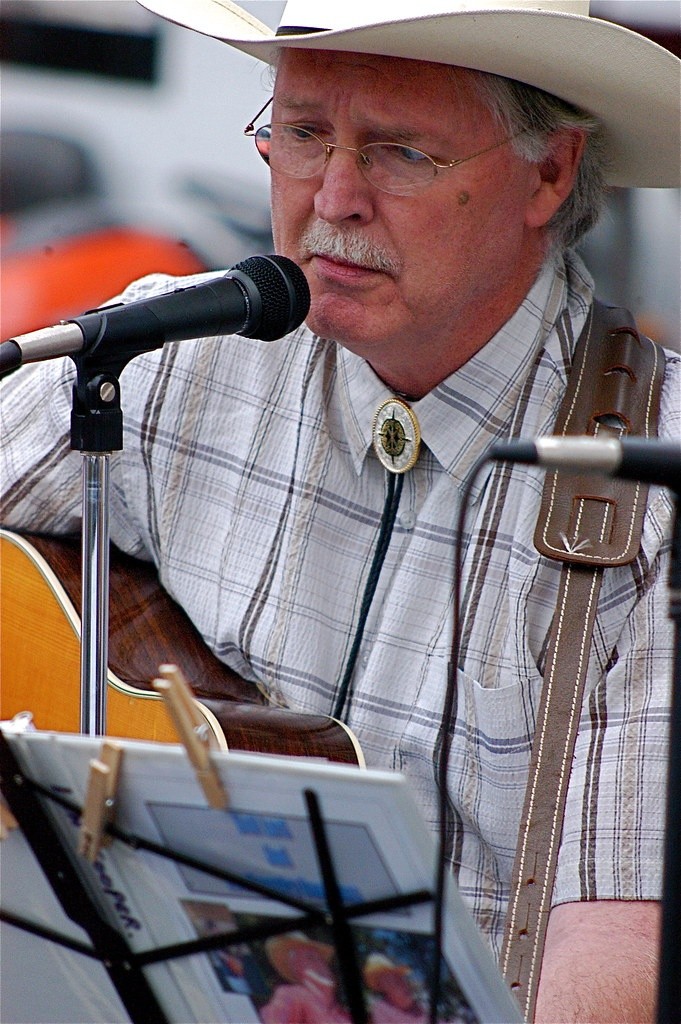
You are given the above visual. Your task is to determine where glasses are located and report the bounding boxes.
[242,98,536,196]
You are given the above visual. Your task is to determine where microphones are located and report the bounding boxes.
[490,432,681,494]
[0,256,312,381]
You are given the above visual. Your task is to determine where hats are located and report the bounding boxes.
[134,0,681,190]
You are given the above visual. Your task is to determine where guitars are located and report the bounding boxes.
[1,527,372,778]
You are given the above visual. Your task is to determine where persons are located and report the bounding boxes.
[0,0,681,1024]
